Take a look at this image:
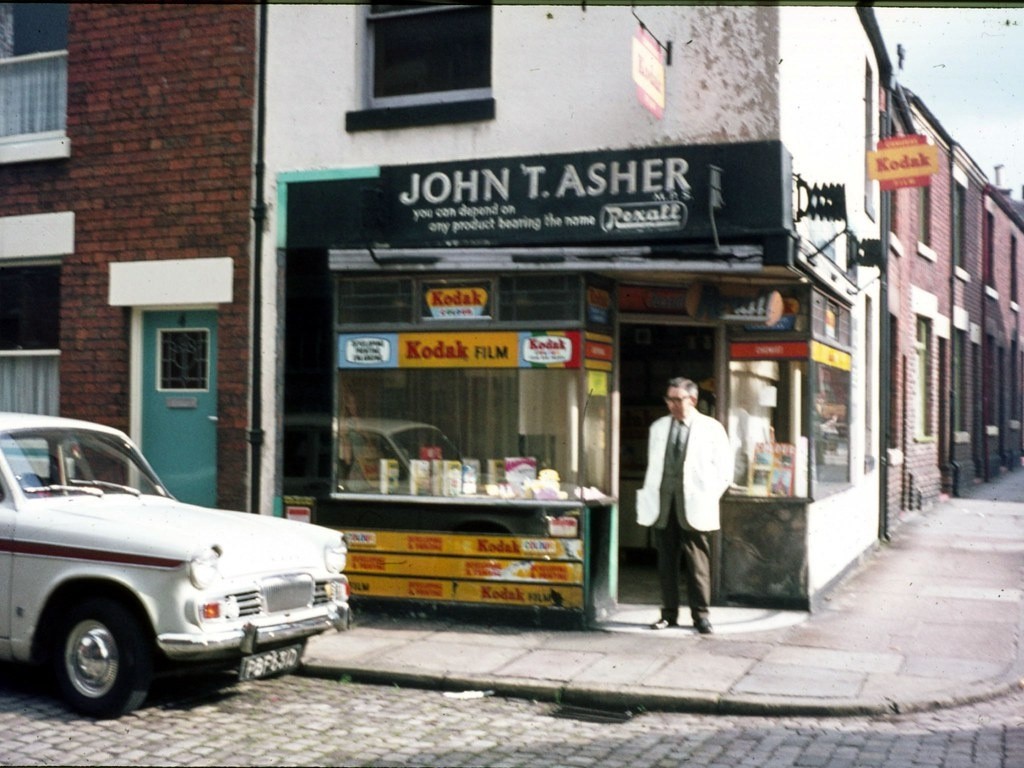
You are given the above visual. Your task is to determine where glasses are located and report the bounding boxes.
[663,395,691,403]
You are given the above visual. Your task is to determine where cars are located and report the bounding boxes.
[0,409,358,722]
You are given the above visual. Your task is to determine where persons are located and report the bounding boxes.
[635,377,734,633]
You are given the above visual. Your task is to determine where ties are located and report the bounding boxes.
[673,421,683,462]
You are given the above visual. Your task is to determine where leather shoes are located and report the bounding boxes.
[651,619,670,629]
[694,618,714,633]
